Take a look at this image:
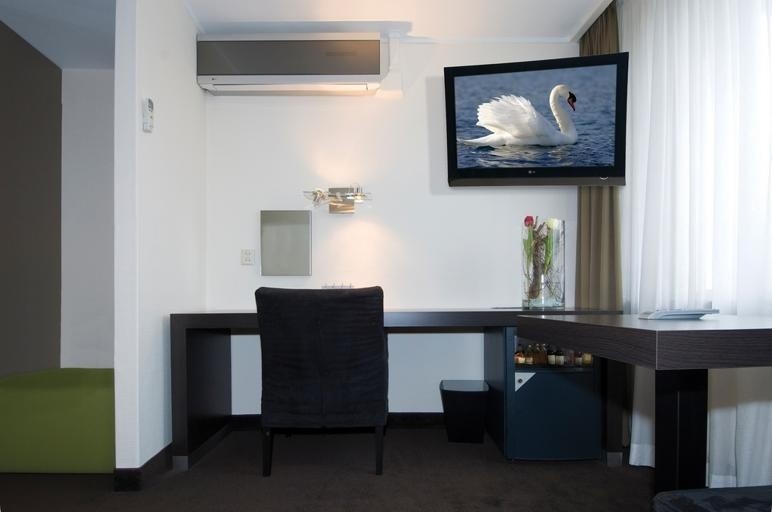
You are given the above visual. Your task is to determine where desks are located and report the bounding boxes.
[169,311,771,496]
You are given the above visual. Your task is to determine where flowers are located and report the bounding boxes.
[521,215,561,300]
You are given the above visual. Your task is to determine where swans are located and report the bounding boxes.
[456,82,579,150]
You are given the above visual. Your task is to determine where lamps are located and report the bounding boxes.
[303,187,372,213]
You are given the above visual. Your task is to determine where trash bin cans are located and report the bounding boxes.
[439,380,489,444]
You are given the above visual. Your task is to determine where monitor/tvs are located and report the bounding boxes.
[444,51,629,188]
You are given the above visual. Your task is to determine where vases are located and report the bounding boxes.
[522,222,566,311]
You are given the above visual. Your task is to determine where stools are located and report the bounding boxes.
[0,367,114,478]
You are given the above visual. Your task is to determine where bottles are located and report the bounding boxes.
[514,343,595,366]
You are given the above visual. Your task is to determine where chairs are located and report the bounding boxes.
[256,287,388,476]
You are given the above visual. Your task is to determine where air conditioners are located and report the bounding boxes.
[196,31,390,94]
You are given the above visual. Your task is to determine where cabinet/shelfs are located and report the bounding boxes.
[483,324,609,465]
